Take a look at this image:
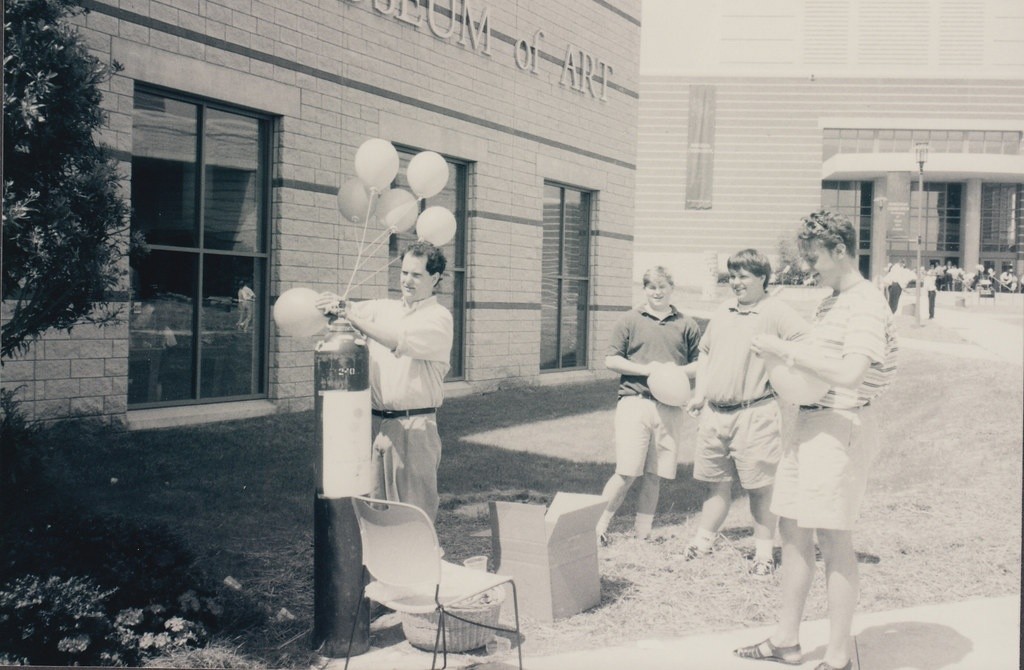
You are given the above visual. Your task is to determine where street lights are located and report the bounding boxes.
[911,139,929,320]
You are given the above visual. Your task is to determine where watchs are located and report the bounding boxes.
[337,297,346,317]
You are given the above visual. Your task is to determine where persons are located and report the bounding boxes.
[236,278,255,333]
[596,265,700,542]
[316,242,454,529]
[920,262,1024,293]
[733,210,898,670]
[688,249,804,574]
[883,260,937,320]
[130,293,181,401]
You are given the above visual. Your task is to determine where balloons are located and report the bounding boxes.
[764,341,833,404]
[338,177,376,223]
[417,207,457,247]
[407,151,448,198]
[648,362,690,407]
[356,138,400,190]
[377,188,418,234]
[272,289,327,337]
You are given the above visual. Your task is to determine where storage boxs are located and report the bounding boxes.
[487,491,610,620]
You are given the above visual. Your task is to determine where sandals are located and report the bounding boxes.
[733,638,804,665]
[815,659,852,670]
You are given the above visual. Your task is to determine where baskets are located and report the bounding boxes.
[400,585,506,653]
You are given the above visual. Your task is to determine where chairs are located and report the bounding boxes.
[343,495,522,670]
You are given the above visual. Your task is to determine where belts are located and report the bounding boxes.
[710,392,779,412]
[372,408,436,418]
[637,392,659,400]
[799,404,832,410]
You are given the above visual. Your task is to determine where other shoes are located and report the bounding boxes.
[633,533,676,546]
[369,598,398,623]
[597,532,607,547]
[675,543,714,562]
[749,556,774,580]
